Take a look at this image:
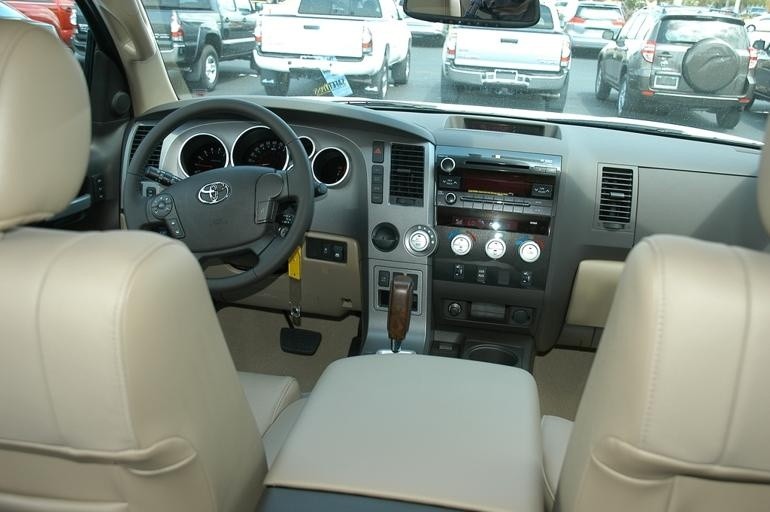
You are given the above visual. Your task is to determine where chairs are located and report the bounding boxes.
[543,111,770,512]
[0,20,300,512]
